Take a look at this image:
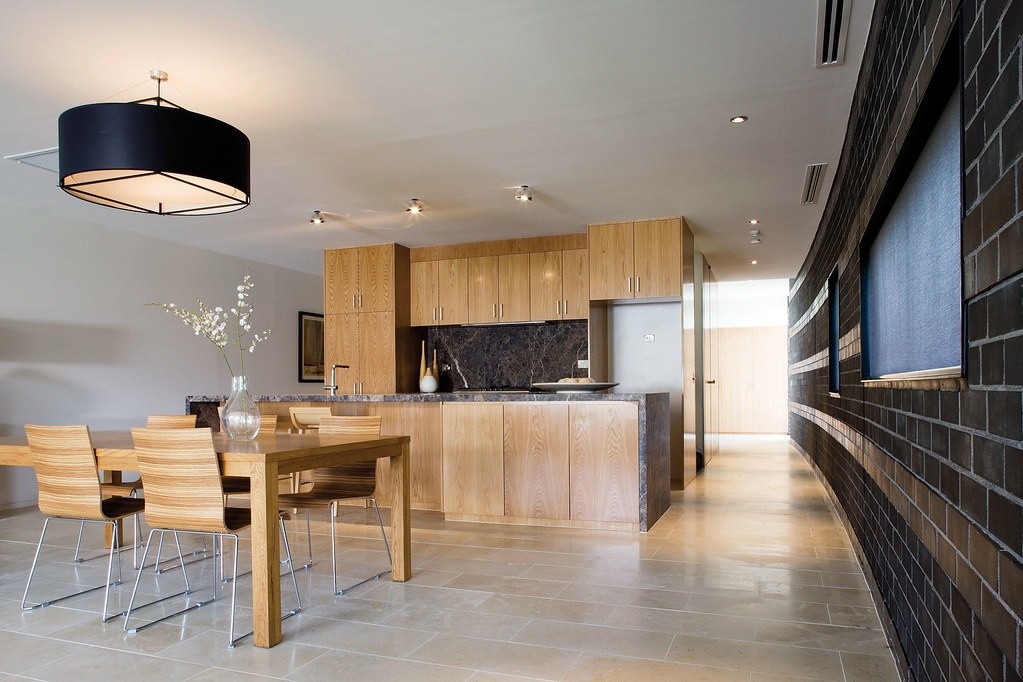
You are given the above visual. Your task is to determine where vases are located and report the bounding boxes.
[227,376,261,441]
[220,375,239,440]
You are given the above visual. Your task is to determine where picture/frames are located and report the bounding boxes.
[297,311,324,384]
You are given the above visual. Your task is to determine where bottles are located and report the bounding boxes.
[419,340,439,393]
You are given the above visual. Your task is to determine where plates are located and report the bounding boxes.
[533,382,620,394]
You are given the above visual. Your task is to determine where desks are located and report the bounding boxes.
[0,430,412,649]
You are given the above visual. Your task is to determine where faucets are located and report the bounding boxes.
[323,364,350,396]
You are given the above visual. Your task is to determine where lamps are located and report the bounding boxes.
[311,211,325,225]
[515,185,532,202]
[58,71,252,215]
[406,199,423,211]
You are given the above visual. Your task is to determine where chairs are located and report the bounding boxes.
[279,416,393,595]
[21,424,190,622]
[74,415,206,568]
[123,427,303,649]
[155,414,291,583]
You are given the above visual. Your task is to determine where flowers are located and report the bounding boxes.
[143,274,272,376]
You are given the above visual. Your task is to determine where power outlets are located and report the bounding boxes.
[577,360,588,369]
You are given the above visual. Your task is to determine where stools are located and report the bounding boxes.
[290,407,368,517]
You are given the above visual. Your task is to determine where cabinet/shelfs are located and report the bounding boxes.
[323,243,396,395]
[588,215,682,300]
[410,249,588,327]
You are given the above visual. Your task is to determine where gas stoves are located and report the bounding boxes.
[453,385,530,393]
